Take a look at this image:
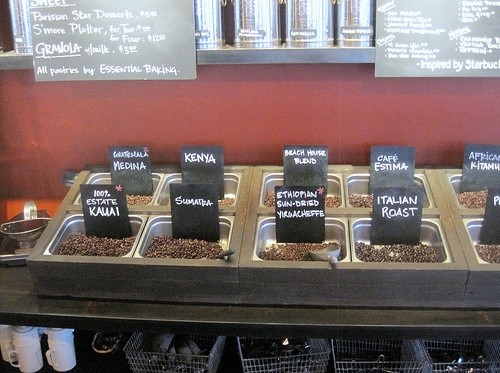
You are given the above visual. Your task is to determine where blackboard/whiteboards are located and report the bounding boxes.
[170,183,222,241]
[478,187,500,245]
[282,144,329,195]
[369,145,417,195]
[370,186,425,246]
[109,145,155,195]
[79,182,133,238]
[28,0,198,81]
[274,184,326,243]
[374,1,499,78]
[460,143,499,191]
[178,144,224,201]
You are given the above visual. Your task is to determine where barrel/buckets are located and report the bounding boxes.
[231,0,284,48]
[337,0,376,49]
[283,0,336,49]
[194,0,227,50]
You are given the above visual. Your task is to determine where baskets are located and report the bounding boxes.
[236,334,331,373]
[416,337,500,373]
[122,329,225,373]
[331,336,426,373]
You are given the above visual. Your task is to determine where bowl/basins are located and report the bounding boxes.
[165,334,208,370]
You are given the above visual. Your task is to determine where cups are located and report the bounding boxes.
[44,327,77,371]
[0,218,52,249]
[0,324,17,362]
[8,326,43,373]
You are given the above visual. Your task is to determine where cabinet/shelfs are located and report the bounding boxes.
[0,44,500,373]
